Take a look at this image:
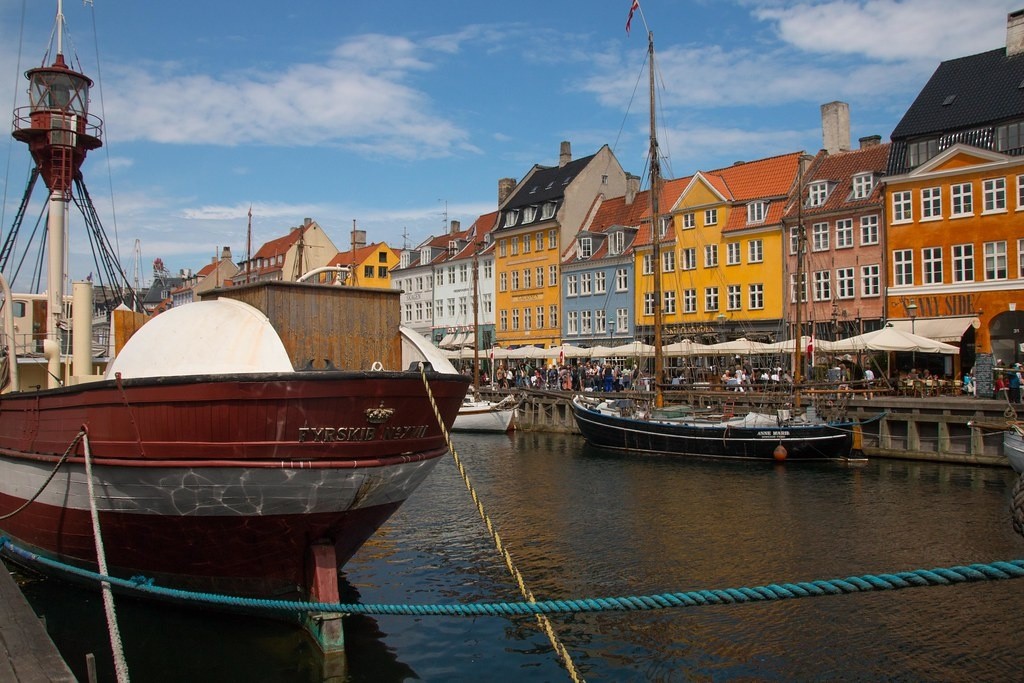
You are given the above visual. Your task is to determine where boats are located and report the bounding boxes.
[1003,428,1024,475]
[0,0,472,646]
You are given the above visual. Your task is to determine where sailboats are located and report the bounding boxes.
[570,0,869,465]
[450,251,527,433]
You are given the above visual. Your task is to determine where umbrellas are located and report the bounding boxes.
[477,326,961,357]
[439,348,475,359]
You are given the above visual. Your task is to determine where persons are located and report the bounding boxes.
[461,358,1024,404]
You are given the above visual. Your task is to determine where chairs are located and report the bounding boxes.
[462,372,964,402]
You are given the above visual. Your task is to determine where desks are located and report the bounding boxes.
[647,407,718,427]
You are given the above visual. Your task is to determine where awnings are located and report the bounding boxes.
[439,333,475,346]
[884,317,981,343]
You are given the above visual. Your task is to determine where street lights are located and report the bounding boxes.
[716,313,727,343]
[608,315,616,347]
[907,299,917,368]
